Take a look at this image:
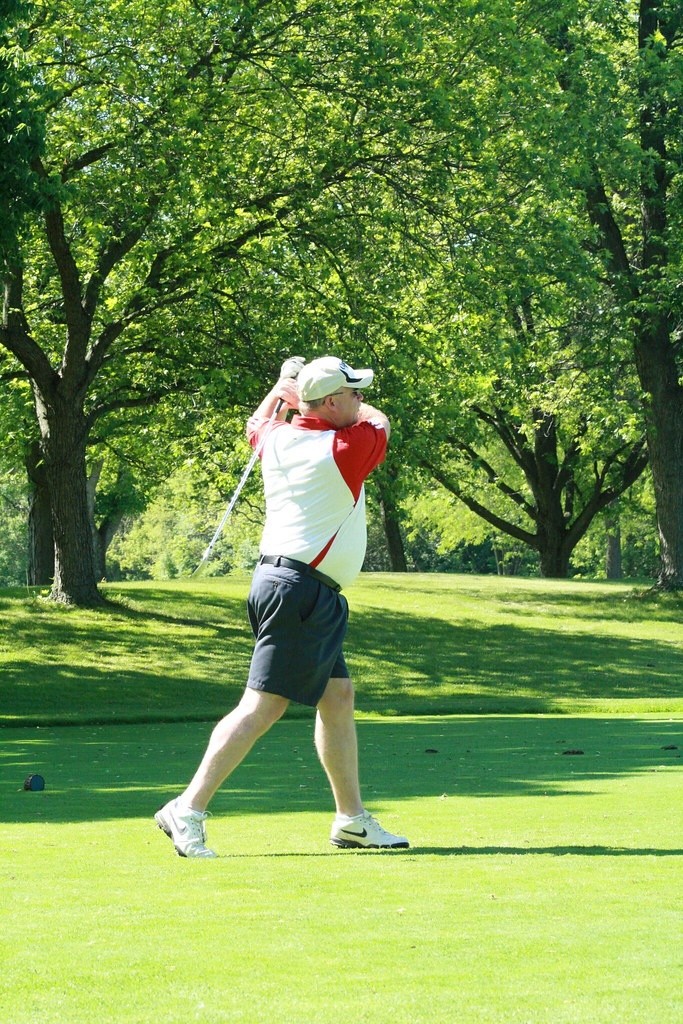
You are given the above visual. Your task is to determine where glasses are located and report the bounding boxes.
[322,390,360,406]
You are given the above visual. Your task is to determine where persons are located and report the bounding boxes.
[154,357,409,859]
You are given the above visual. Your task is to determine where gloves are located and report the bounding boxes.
[279,356,306,385]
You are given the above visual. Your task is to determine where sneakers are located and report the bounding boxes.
[329,809,409,848]
[154,796,216,859]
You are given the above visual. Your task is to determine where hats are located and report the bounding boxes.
[296,356,374,401]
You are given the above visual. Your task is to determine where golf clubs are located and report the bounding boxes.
[190,367,295,574]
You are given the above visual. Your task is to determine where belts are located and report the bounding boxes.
[260,555,343,592]
